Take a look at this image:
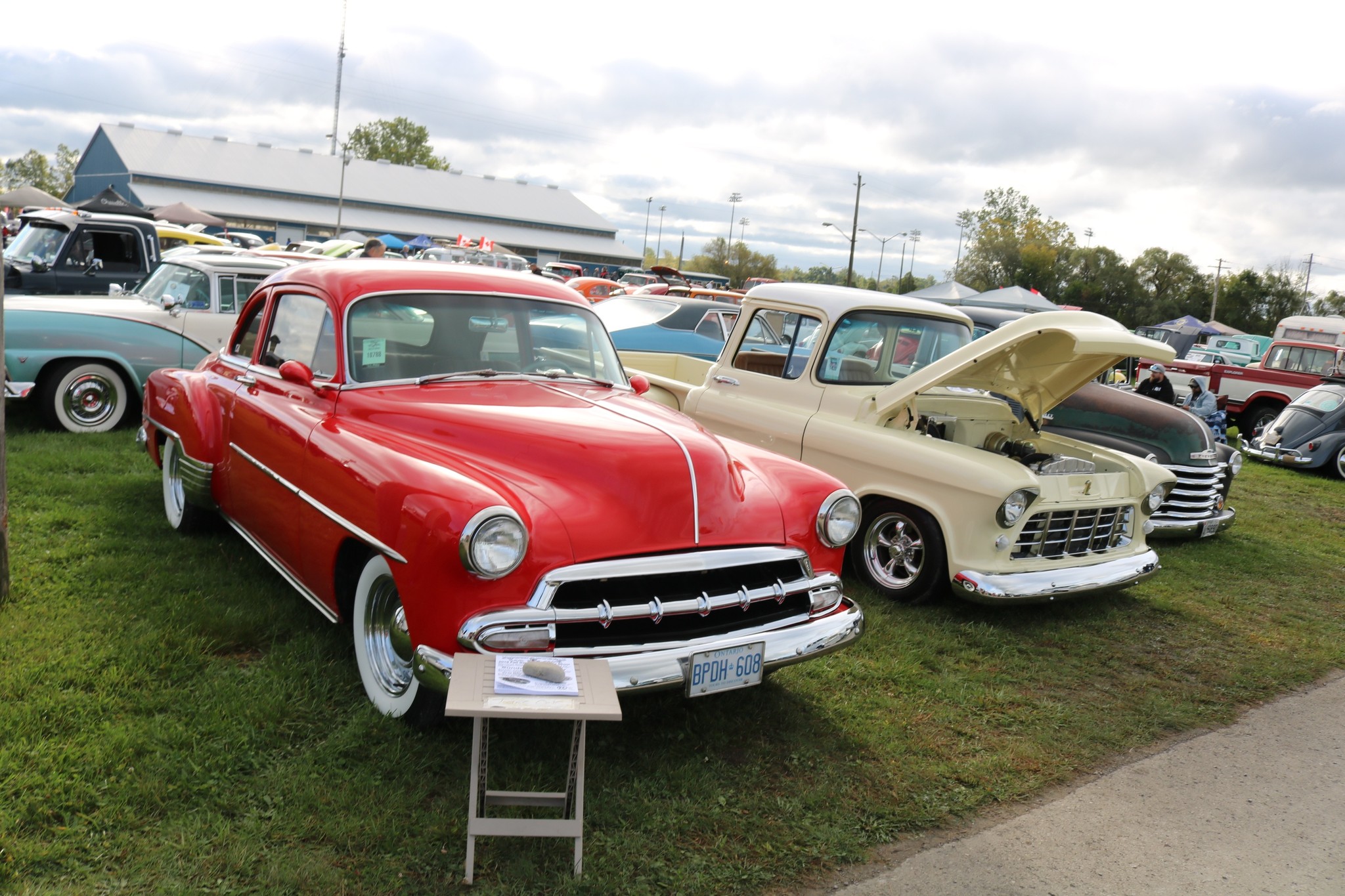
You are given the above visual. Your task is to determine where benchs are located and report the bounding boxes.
[734,351,875,383]
[352,352,454,383]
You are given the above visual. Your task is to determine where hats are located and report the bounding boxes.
[1188,379,1198,386]
[1147,364,1165,373]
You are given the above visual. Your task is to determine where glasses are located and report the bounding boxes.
[1188,384,1199,389]
[1150,365,1157,370]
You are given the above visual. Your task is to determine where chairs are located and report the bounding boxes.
[458,360,522,373]
[1204,395,1228,444]
[1280,358,1293,370]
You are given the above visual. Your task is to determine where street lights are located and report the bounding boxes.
[822,221,856,287]
[1085,228,1094,249]
[738,215,751,244]
[641,196,652,269]
[908,229,921,280]
[656,204,667,266]
[858,228,908,291]
[727,192,743,259]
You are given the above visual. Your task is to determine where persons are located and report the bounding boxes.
[1179,375,1217,418]
[267,235,291,246]
[529,264,543,277]
[1134,363,1175,405]
[360,237,484,267]
[582,265,735,292]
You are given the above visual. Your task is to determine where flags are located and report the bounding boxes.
[479,236,495,252]
[457,234,472,249]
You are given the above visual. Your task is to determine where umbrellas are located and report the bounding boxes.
[960,285,1064,311]
[900,281,981,305]
[1204,320,1249,336]
[405,234,442,249]
[1153,315,1221,335]
[375,234,413,250]
[0,184,226,229]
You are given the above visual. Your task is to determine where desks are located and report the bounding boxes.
[445,652,621,885]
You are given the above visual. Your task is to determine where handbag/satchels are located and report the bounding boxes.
[1205,410,1227,444]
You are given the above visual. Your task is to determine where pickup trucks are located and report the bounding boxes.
[533,269,1181,609]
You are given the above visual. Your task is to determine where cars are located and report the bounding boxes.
[1,203,1345,544]
[2,255,520,434]
[135,256,866,737]
